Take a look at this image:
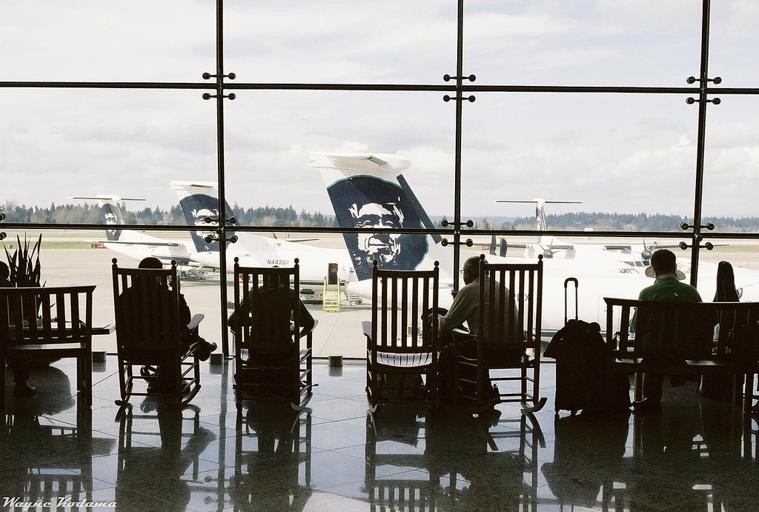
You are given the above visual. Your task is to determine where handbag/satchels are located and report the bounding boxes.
[147,373,190,393]
[698,370,732,401]
[380,372,418,400]
[722,326,759,362]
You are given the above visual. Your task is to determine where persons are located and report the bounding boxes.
[629,249,704,413]
[103,213,117,238]
[428,256,527,408]
[343,201,409,268]
[227,265,314,367]
[118,257,218,394]
[189,208,223,243]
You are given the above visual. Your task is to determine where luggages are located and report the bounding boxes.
[556,277,607,414]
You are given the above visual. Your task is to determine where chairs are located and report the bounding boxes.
[112,254,547,410]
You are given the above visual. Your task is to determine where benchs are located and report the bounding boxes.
[0,285,96,412]
[604,295,758,416]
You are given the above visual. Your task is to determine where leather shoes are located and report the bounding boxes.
[633,397,660,408]
[14,383,37,397]
[200,342,217,362]
